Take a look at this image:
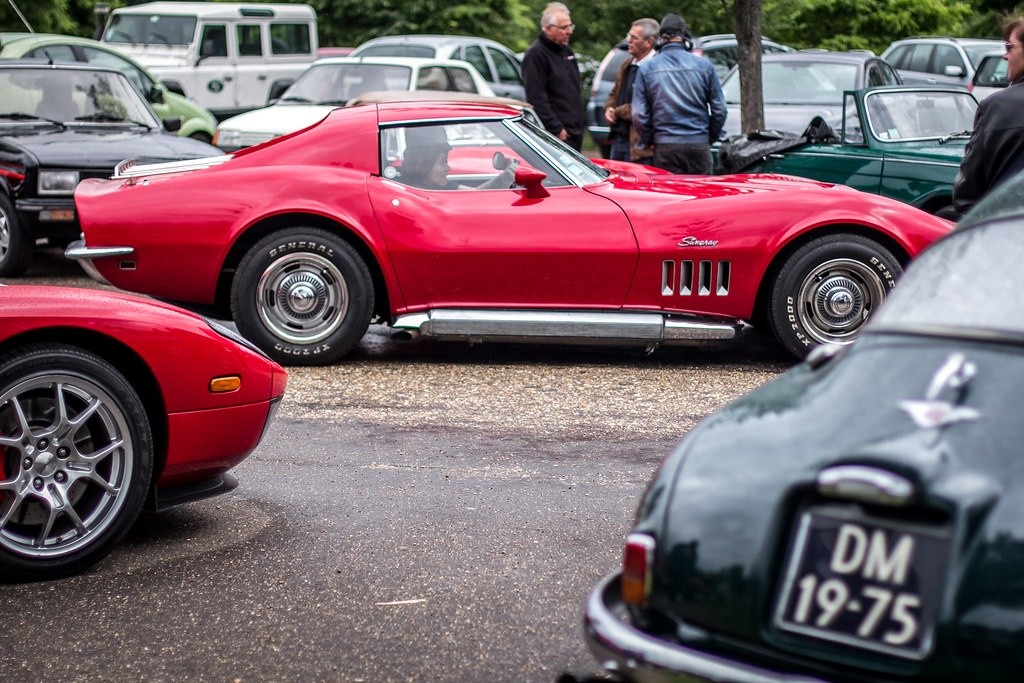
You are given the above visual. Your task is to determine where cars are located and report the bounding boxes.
[212,55,505,159]
[344,34,528,104]
[0,57,227,280]
[0,33,221,146]
[573,33,1012,143]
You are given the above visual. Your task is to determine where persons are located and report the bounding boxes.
[631,13,728,174]
[604,18,660,166]
[952,18,1024,219]
[522,2,584,152]
[401,126,518,191]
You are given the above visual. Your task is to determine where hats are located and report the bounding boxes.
[659,14,691,39]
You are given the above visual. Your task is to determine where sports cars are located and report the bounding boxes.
[708,82,988,224]
[73,96,958,369]
[577,165,1024,683]
[0,277,289,584]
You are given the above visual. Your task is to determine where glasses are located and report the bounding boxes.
[626,32,643,41]
[1005,43,1024,52]
[549,23,575,30]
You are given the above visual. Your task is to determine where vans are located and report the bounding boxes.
[99,1,318,124]
